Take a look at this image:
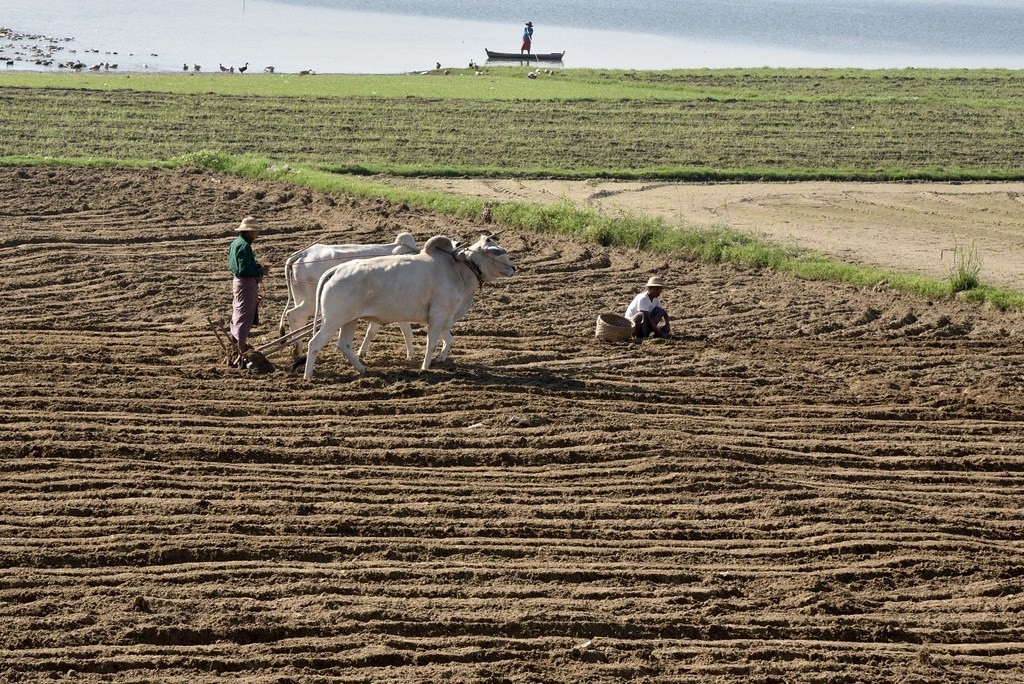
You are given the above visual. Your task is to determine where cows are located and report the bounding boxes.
[279,231,517,381]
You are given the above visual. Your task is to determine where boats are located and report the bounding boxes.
[485,48,566,62]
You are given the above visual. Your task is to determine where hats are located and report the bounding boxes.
[644,277,669,287]
[526,22,534,27]
[235,218,266,232]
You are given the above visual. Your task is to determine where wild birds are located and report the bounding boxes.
[0,22,317,75]
[436,58,559,78]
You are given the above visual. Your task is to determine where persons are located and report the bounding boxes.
[624,277,670,339]
[521,22,533,54]
[229,217,272,360]
[521,59,529,67]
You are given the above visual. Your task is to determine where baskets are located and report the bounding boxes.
[595,313,636,342]
